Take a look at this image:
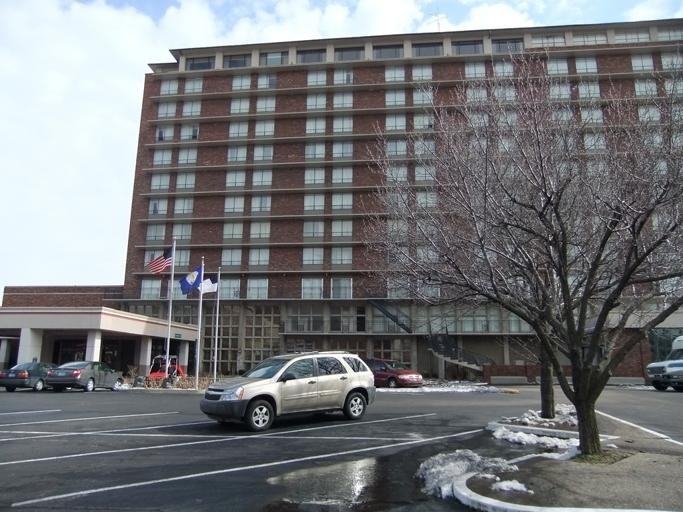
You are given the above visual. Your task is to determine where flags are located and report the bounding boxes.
[196,272,218,295]
[176,265,200,294]
[145,246,171,276]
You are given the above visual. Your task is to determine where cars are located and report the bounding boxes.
[0,362,72,390]
[46,360,126,392]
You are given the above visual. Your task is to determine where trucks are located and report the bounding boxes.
[647,335,683,394]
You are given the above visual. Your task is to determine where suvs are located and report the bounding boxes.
[362,356,423,388]
[198,347,375,432]
